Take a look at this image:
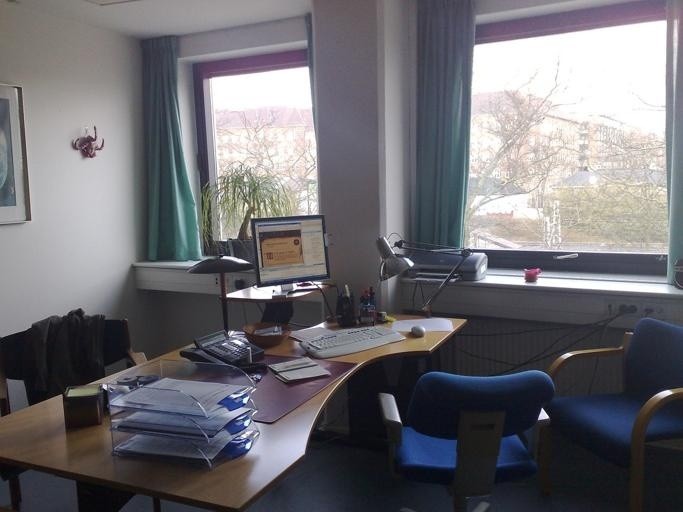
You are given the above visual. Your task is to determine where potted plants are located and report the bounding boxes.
[198,168,293,270]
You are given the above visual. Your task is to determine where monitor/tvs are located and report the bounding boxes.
[251,215,331,300]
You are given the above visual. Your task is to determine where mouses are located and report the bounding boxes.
[411,325,426,337]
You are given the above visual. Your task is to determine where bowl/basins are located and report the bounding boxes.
[242,322,289,348]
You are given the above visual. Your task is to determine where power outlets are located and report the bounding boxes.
[604,299,672,320]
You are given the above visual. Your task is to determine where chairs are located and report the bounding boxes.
[23,318,161,512]
[539,318,683,512]
[370,370,555,511]
[0,339,23,512]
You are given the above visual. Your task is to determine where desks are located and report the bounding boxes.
[0,312,467,512]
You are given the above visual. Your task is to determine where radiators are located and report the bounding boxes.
[419,316,629,458]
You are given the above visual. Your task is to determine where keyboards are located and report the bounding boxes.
[299,326,407,359]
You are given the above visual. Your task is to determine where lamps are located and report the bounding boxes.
[376,231,473,318]
[186,252,256,335]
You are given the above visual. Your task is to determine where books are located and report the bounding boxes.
[107,374,257,470]
[268,356,332,388]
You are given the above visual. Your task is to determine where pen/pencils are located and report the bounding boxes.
[339,285,353,304]
[360,286,375,306]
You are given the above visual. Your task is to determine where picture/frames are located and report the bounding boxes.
[0,79,32,225]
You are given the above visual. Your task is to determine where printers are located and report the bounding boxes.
[407,249,488,286]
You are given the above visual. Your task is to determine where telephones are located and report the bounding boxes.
[180,329,264,365]
